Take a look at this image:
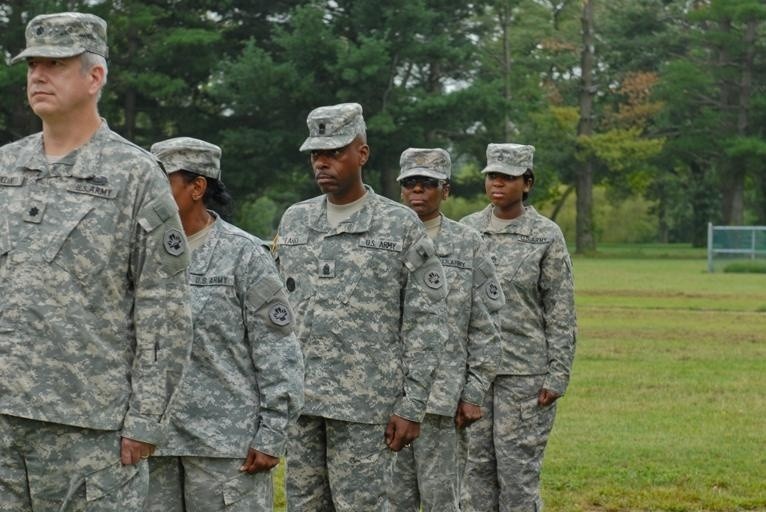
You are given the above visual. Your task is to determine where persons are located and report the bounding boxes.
[0,10,195,511]
[275,99,451,511]
[458,142,579,511]
[147,135,304,511]
[395,144,504,511]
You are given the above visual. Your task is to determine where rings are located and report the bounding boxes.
[142,455,149,458]
[404,443,411,447]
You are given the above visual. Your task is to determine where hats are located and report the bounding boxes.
[10,12,107,64]
[150,137,222,180]
[481,144,535,176]
[396,148,452,182]
[298,103,367,152]
